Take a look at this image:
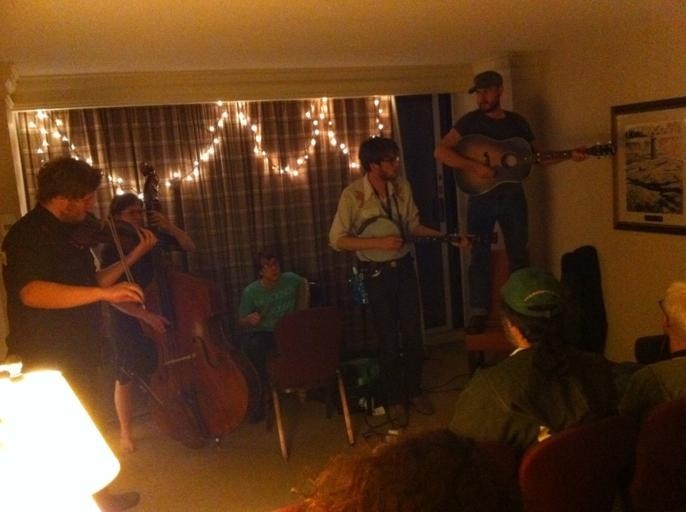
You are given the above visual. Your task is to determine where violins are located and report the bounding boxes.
[68,213,158,254]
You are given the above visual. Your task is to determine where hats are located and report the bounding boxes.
[468,69,503,94]
[501,267,564,318]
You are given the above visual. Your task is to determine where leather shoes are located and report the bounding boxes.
[100,492,139,512]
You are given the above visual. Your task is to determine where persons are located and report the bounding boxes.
[617,278,686,416]
[101,192,195,453]
[431,68,591,333]
[0,157,158,511]
[448,266,617,442]
[327,135,448,427]
[236,250,303,332]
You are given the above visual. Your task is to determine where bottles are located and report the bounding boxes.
[351,265,371,305]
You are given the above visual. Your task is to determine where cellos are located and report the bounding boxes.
[136,162,261,446]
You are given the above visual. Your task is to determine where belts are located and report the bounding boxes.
[372,257,406,270]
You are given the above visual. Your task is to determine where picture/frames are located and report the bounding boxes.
[611,96,686,237]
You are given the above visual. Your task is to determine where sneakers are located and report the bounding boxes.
[389,404,408,427]
[409,395,433,415]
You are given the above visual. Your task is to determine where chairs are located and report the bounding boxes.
[518,415,640,511]
[463,249,516,379]
[295,278,311,311]
[629,399,686,511]
[265,307,355,462]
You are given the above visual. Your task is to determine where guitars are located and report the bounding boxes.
[453,132,617,195]
